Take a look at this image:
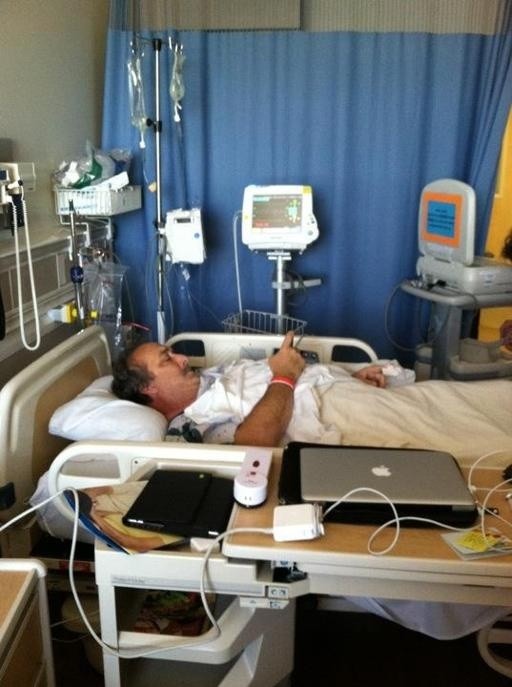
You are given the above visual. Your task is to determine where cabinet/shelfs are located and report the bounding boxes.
[0,557,56,687]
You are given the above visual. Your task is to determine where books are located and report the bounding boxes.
[440,528,512,560]
[63,479,191,556]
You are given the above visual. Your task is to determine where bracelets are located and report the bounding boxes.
[271,377,296,391]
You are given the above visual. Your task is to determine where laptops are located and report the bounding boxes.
[299,447,476,516]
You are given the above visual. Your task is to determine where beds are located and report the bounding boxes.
[0,324,512,687]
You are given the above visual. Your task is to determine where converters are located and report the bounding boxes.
[273,504,322,541]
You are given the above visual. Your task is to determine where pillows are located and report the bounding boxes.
[48,375,168,443]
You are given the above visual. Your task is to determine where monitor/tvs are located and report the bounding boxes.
[420,193,461,247]
[242,184,313,250]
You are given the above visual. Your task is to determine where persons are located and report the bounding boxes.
[82,481,185,553]
[112,329,386,447]
